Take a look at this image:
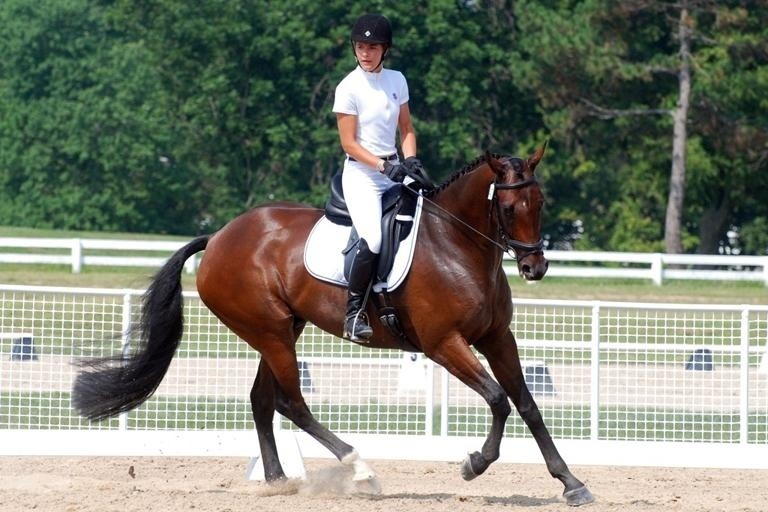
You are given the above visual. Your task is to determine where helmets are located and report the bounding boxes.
[351,13,392,46]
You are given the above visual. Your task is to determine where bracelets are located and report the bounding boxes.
[375,160,385,173]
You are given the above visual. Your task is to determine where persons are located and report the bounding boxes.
[331,13,432,345]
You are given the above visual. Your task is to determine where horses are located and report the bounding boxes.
[68,139,596,507]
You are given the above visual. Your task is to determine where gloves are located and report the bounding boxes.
[379,161,408,183]
[402,156,423,175]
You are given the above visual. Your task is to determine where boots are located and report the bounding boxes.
[343,237,383,341]
